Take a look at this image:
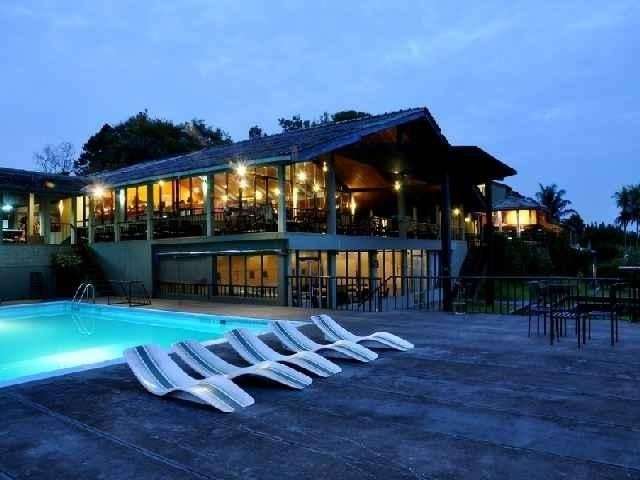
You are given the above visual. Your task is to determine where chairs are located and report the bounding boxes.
[582,285,618,346]
[528,281,552,338]
[548,284,580,350]
[309,313,415,353]
[124,344,256,414]
[269,319,379,364]
[223,327,343,379]
[172,339,314,391]
